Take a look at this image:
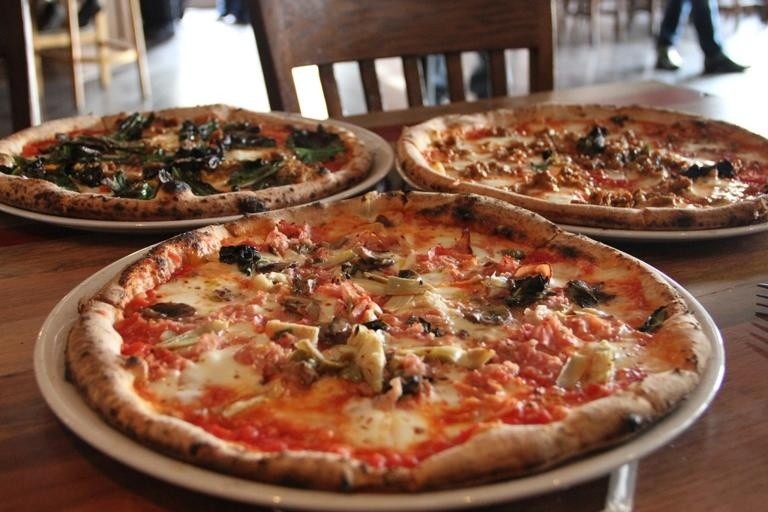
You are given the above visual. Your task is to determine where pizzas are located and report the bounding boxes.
[0,103,374,221]
[398,103,768,231]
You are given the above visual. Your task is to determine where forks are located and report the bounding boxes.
[755,283,767,319]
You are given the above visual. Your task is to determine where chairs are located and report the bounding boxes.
[248,0,553,118]
[555,2,744,46]
[33,0,153,110]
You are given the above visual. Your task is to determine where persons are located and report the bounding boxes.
[656,0,751,74]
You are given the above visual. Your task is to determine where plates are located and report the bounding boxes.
[398,108,764,250]
[0,105,395,236]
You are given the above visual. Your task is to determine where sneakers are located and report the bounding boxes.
[705,49,750,73]
[657,47,679,70]
